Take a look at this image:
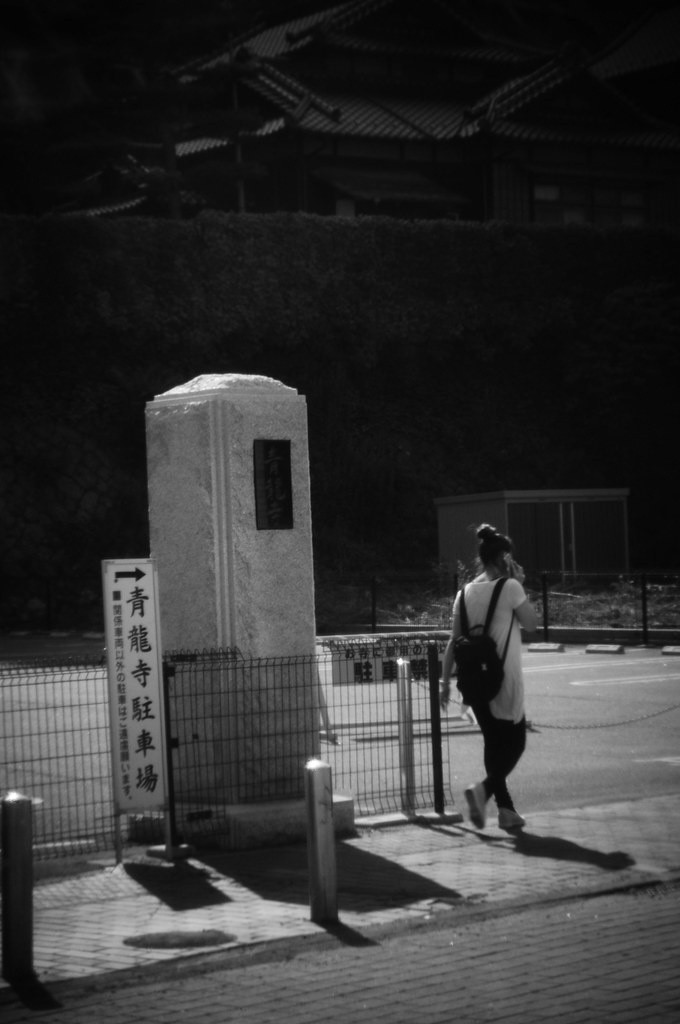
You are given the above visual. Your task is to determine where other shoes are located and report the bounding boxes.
[465,781,487,828]
[498,807,525,829]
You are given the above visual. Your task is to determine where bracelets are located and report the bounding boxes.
[439,679,451,686]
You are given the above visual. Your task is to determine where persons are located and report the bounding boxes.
[440,523,538,828]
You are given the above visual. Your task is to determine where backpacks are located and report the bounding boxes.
[452,577,515,705]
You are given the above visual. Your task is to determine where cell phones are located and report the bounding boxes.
[504,553,513,566]
